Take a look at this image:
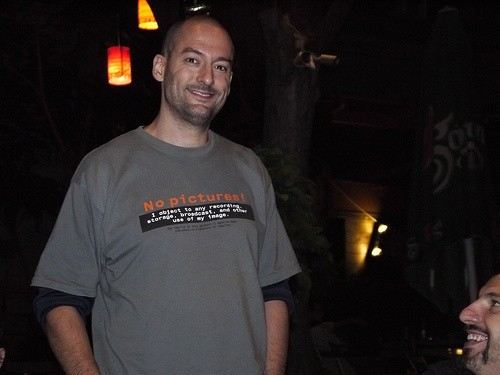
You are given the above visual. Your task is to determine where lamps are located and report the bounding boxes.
[137,0,159,30]
[107,31,132,86]
[365,222,389,265]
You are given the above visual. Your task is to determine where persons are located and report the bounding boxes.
[459,273,500,375]
[29,16,302,375]
